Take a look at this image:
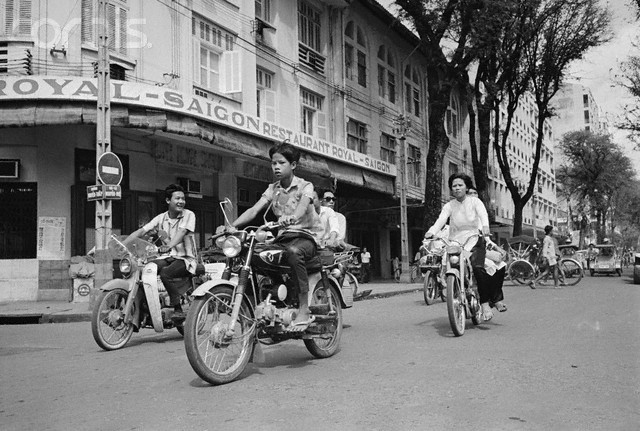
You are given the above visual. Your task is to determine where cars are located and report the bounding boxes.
[589,244,626,275]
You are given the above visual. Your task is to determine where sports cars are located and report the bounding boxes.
[316,241,354,311]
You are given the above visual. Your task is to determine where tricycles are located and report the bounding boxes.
[507,236,583,287]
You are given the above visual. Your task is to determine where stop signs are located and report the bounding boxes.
[97,152,123,186]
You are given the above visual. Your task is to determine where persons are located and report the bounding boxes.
[465,186,508,312]
[538,231,569,287]
[117,183,198,320]
[422,211,451,300]
[425,173,494,323]
[528,225,562,290]
[319,189,345,291]
[215,140,326,335]
[359,248,373,283]
[309,187,337,252]
[583,234,590,249]
[586,245,596,266]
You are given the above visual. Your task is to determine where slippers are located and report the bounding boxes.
[285,312,316,331]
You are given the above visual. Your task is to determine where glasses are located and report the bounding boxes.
[323,195,336,202]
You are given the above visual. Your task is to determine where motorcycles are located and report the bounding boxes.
[426,233,483,337]
[184,189,352,385]
[421,246,447,305]
[91,231,207,351]
